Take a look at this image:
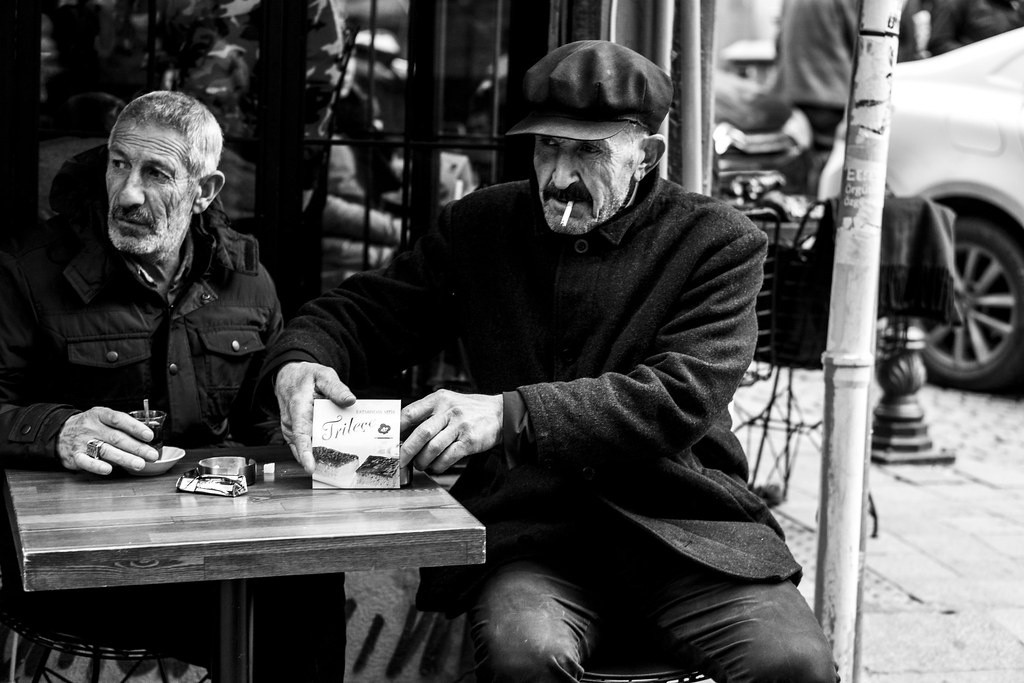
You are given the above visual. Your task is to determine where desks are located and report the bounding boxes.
[4,442,487,682]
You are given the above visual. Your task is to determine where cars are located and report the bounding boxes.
[816,23,1024,391]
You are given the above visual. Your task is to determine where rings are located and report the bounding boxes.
[86,439,105,459]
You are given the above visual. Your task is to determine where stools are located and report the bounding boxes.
[576,617,710,683]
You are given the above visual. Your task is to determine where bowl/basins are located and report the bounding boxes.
[126,446,186,476]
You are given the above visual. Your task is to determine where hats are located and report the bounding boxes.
[505,40,672,141]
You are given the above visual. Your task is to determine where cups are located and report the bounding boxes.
[130,409,168,460]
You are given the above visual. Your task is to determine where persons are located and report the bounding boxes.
[156,0,346,356]
[267,40,847,683]
[740,0,1023,369]
[0,89,350,683]
